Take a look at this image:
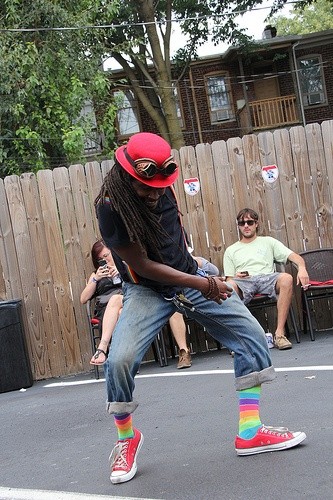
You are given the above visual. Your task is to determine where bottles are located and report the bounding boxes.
[263,329,273,349]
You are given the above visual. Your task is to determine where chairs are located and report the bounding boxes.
[290,248,333,341]
[85,299,221,379]
[245,293,300,344]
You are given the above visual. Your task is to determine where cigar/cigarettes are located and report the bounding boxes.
[300,285,310,287]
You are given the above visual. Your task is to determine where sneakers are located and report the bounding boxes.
[234,424,308,456]
[108,428,144,485]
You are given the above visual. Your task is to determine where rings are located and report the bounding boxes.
[224,293,227,299]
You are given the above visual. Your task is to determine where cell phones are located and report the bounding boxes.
[241,271,249,277]
[98,259,108,272]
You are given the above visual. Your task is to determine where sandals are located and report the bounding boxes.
[89,347,109,365]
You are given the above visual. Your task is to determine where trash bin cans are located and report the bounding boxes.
[0,298,36,393]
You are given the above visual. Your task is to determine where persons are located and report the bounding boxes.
[94,133,307,484]
[169,227,219,370]
[80,239,124,365]
[223,208,310,356]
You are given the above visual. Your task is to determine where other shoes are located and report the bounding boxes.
[177,347,193,370]
[274,335,293,350]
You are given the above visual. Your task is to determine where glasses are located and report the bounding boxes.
[97,252,111,262]
[123,149,179,179]
[237,219,257,226]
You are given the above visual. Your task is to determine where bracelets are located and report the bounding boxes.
[93,277,97,282]
[201,276,220,301]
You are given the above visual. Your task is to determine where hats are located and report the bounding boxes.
[114,132,180,190]
